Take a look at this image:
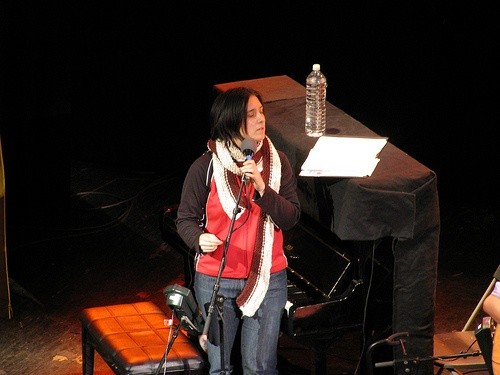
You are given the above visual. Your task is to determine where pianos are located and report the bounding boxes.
[212,74,440,375]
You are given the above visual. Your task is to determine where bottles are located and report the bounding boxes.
[304,63,327,137]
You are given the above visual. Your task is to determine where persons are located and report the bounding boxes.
[483,281,500,375]
[177,83,300,375]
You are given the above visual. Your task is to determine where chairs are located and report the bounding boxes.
[430,262,500,375]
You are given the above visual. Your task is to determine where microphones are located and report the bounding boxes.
[241,137,258,184]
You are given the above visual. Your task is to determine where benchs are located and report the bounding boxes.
[81,298,206,375]
